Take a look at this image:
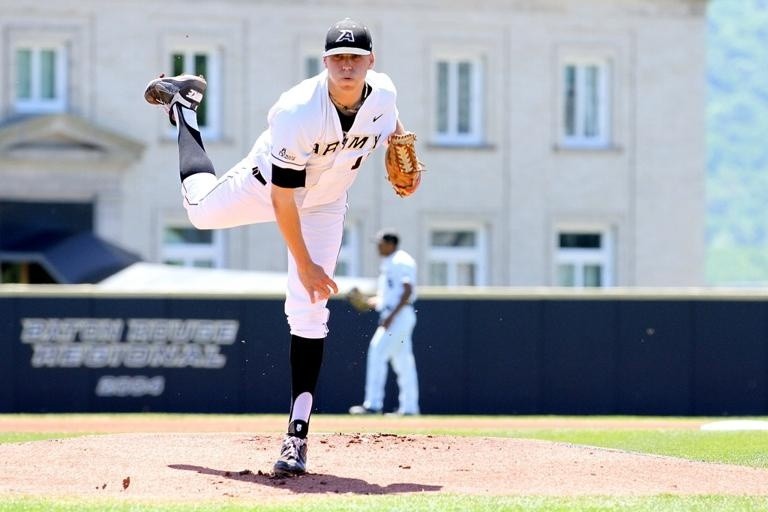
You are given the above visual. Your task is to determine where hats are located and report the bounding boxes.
[322,17,372,58]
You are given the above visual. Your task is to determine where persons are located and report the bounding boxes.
[145,17,419,488]
[347,234,423,416]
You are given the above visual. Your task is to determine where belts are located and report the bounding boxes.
[250,166,267,186]
[383,303,415,312]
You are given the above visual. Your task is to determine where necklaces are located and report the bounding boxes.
[331,84,368,114]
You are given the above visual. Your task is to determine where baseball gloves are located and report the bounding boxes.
[384,131,422,201]
[347,287,370,312]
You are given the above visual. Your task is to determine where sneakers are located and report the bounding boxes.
[272,433,308,478]
[143,73,208,127]
[349,405,384,416]
[383,410,420,417]
